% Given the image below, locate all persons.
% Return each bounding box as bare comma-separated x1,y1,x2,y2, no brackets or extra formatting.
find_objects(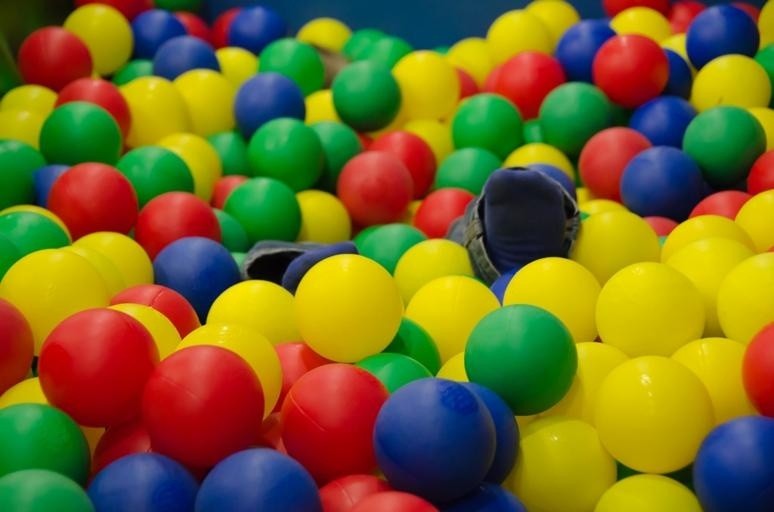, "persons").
242,166,581,289
